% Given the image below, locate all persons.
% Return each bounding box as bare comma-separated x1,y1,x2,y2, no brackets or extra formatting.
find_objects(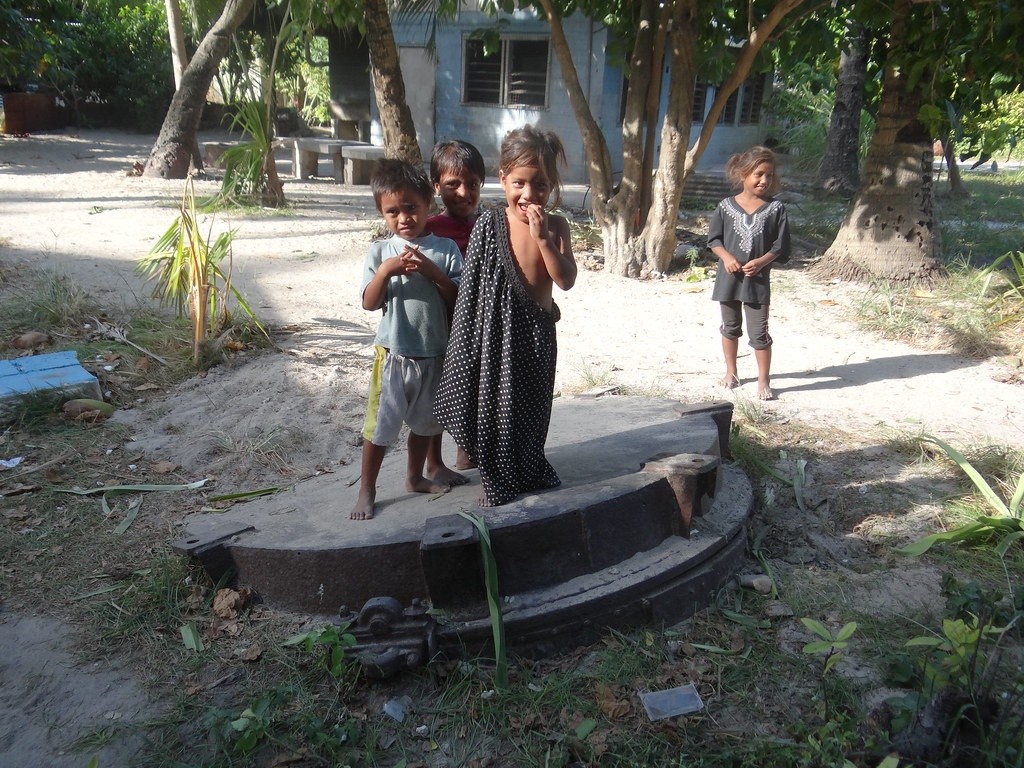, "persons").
431,124,578,506
347,138,487,520
706,146,791,401
